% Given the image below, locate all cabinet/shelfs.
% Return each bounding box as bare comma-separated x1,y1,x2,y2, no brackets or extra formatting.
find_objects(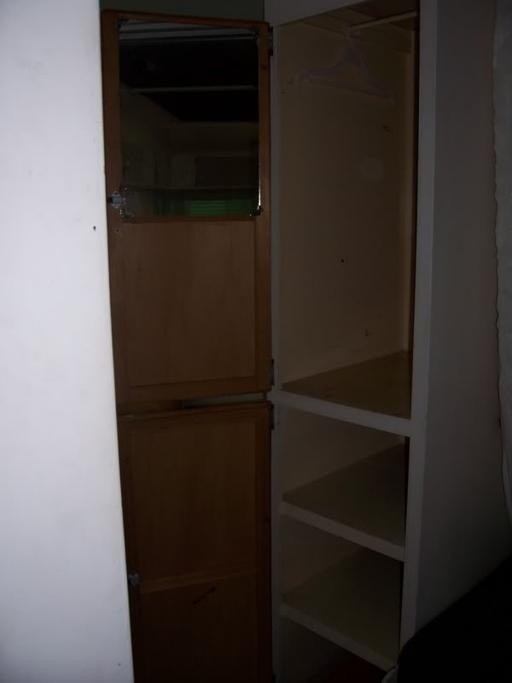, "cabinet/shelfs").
100,19,497,659
